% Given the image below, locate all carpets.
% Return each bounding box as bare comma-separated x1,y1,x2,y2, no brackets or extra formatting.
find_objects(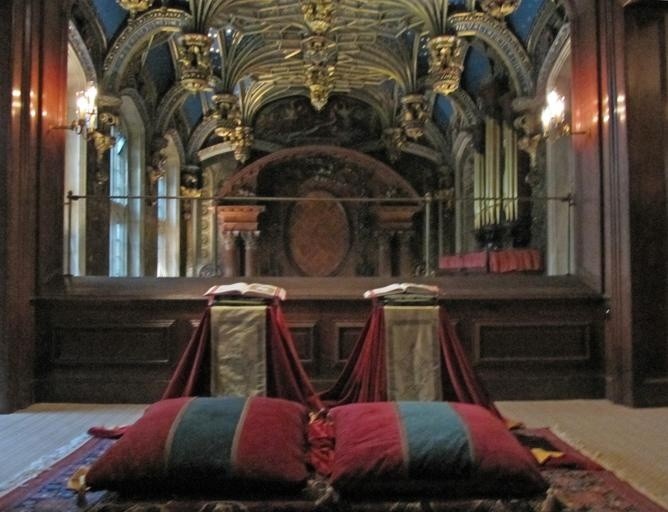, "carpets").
1,426,667,511
